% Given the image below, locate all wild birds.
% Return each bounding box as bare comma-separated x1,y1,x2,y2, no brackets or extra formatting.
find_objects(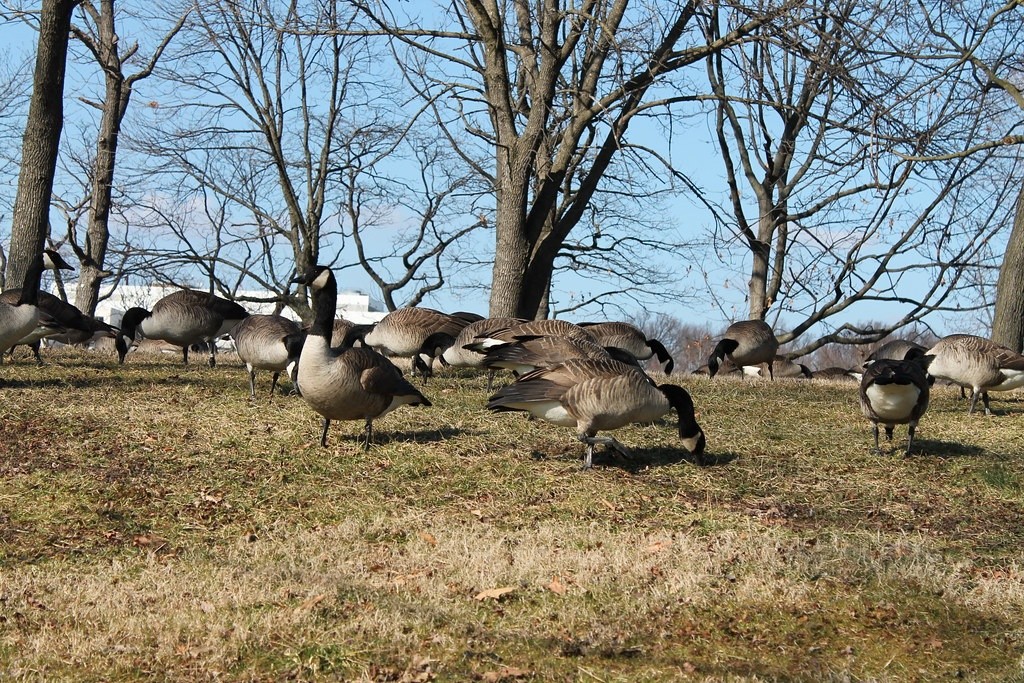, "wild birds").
40,316,122,351
0,288,140,369
0,248,76,366
689,338,937,389
926,336,1024,417
112,288,675,406
859,354,937,461
484,357,707,468
287,265,433,454
707,320,780,382
923,334,982,402
